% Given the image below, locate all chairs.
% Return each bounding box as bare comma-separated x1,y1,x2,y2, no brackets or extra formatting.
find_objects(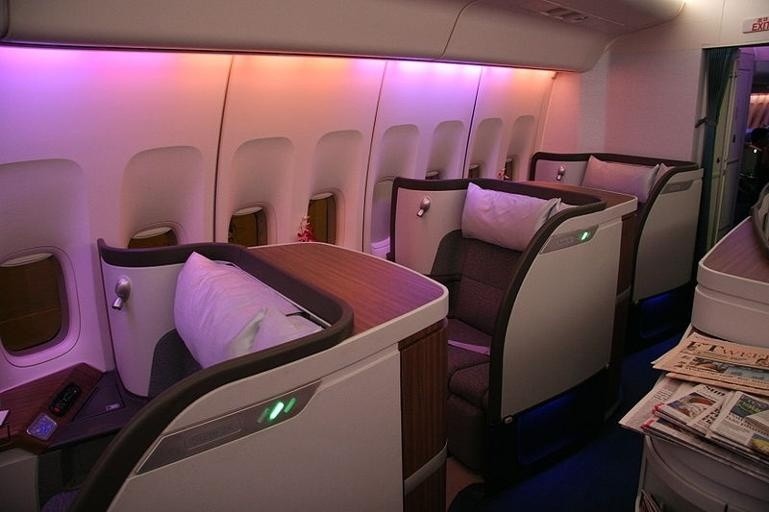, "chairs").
388,150,703,475
1,238,447,512
